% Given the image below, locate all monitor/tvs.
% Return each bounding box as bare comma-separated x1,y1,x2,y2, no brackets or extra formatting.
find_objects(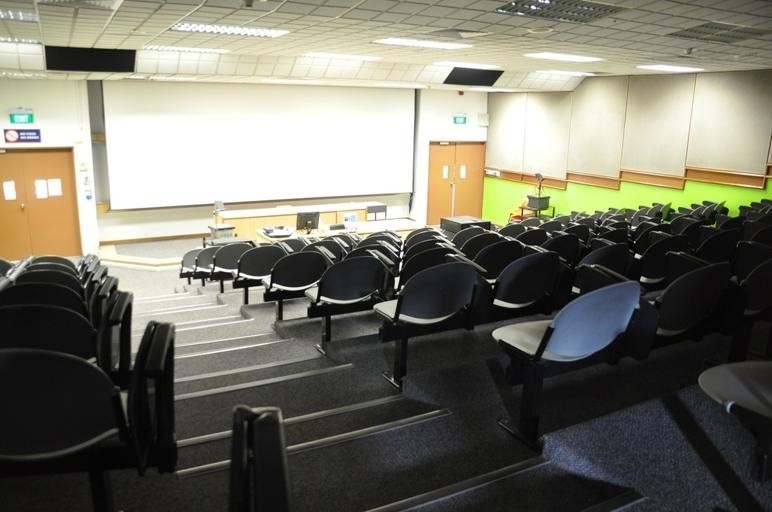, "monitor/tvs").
297,212,320,234
